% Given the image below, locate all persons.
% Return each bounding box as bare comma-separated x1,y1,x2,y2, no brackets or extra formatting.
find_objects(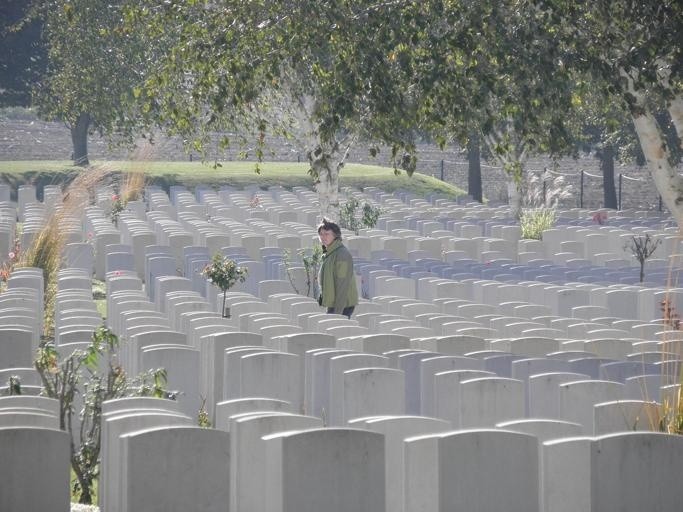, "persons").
317,221,358,319
